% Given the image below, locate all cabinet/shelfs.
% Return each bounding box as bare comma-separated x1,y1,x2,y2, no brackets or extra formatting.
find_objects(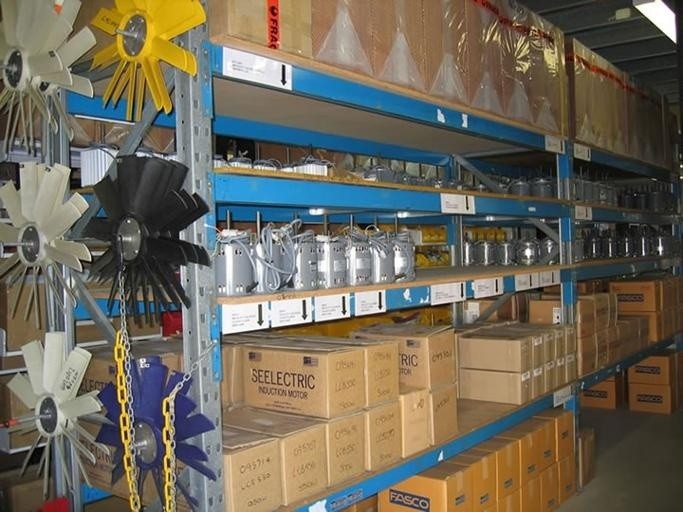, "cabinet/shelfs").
0,0,682,512
